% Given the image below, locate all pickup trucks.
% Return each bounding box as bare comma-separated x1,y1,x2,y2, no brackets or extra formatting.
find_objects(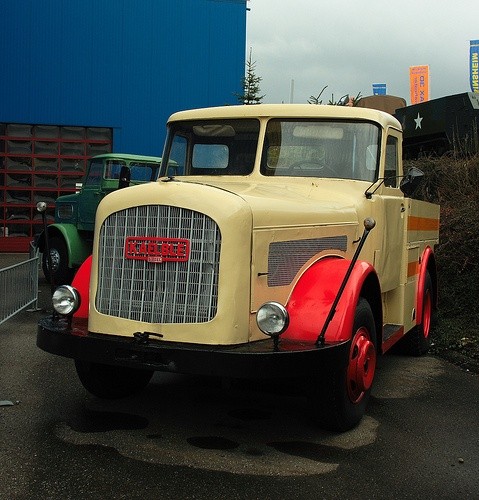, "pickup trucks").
37,104,441,435
36,153,177,288
